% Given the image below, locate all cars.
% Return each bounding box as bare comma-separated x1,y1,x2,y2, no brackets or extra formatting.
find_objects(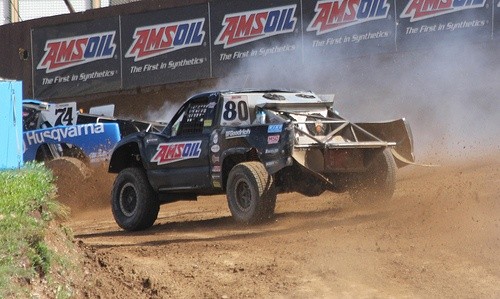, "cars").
108,88,440,233
